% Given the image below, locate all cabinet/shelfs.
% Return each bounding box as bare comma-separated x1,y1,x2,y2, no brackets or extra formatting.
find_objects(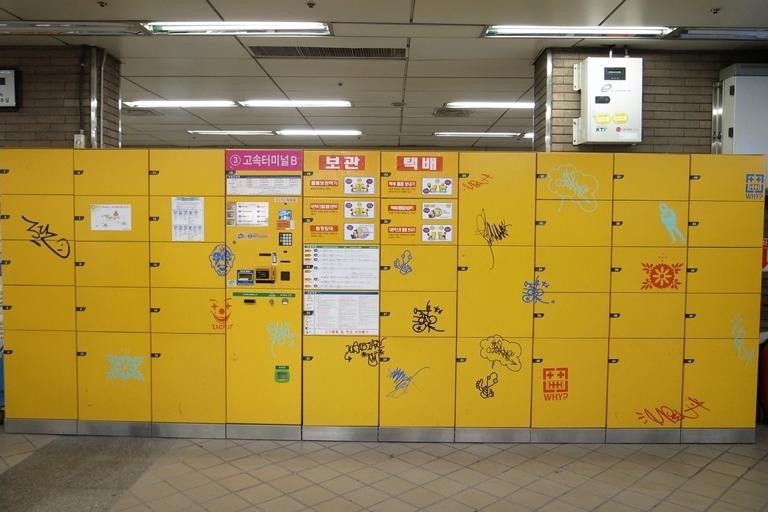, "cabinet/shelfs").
0,145,767,443
